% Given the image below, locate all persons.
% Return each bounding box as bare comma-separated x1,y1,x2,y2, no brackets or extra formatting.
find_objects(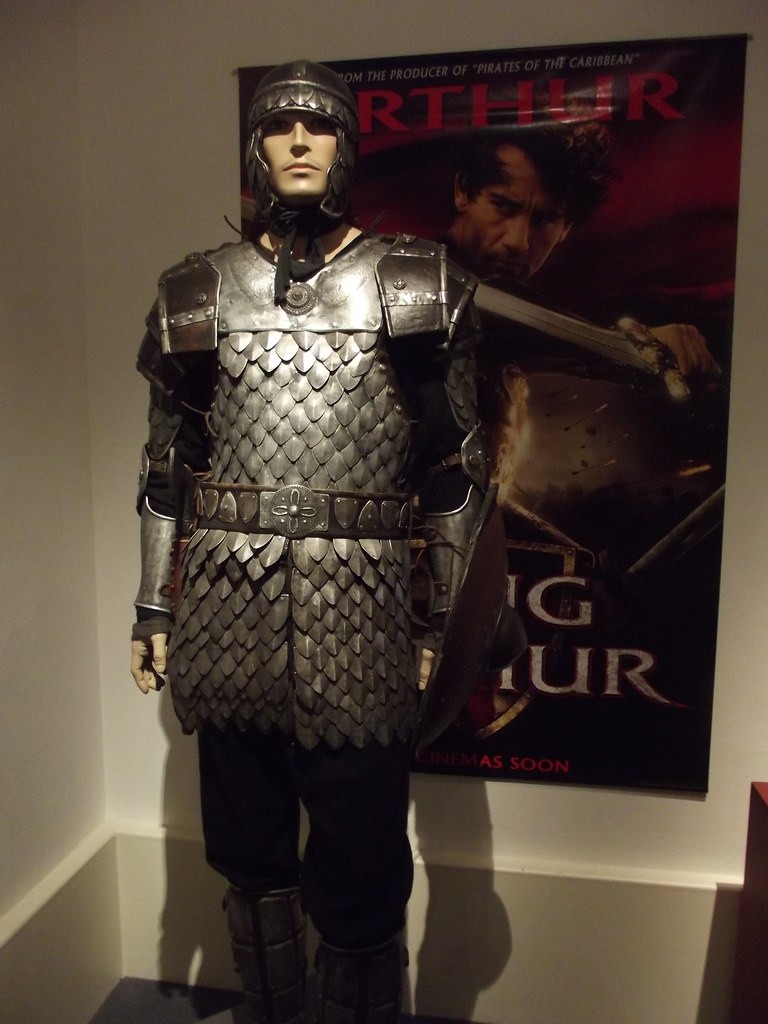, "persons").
426,106,714,776
128,59,493,1024
